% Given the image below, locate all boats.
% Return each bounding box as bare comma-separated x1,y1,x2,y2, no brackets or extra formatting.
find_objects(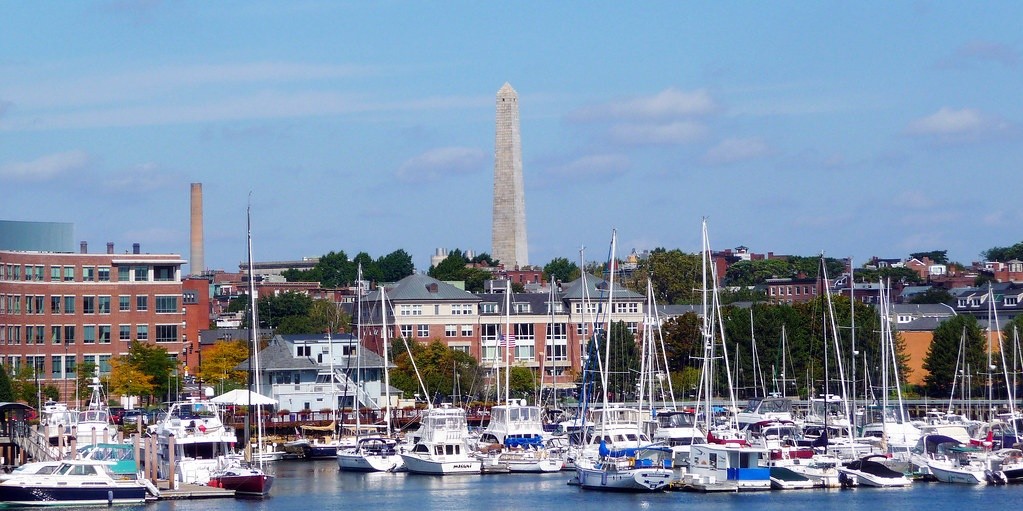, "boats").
286,216,1023,492
40,374,144,460
0,447,158,506
124,398,275,500
